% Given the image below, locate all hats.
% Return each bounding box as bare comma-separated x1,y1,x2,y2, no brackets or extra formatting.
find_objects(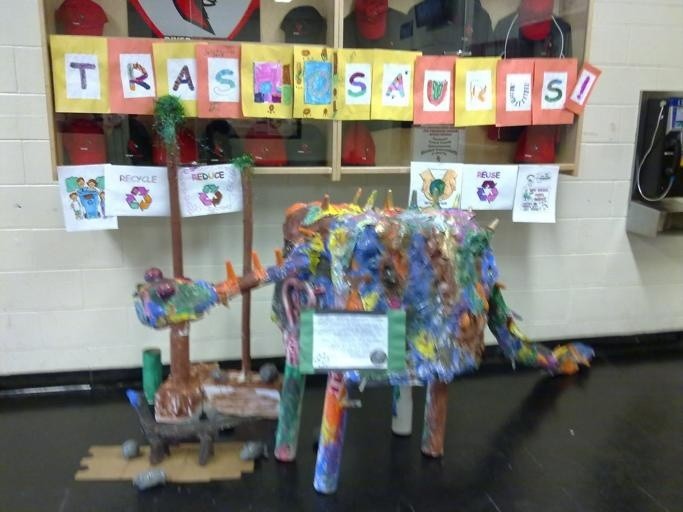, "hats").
63,120,327,166
519,1,553,40
355,0,388,40
342,124,375,165
513,126,556,162
280,6,327,44
55,0,108,36
